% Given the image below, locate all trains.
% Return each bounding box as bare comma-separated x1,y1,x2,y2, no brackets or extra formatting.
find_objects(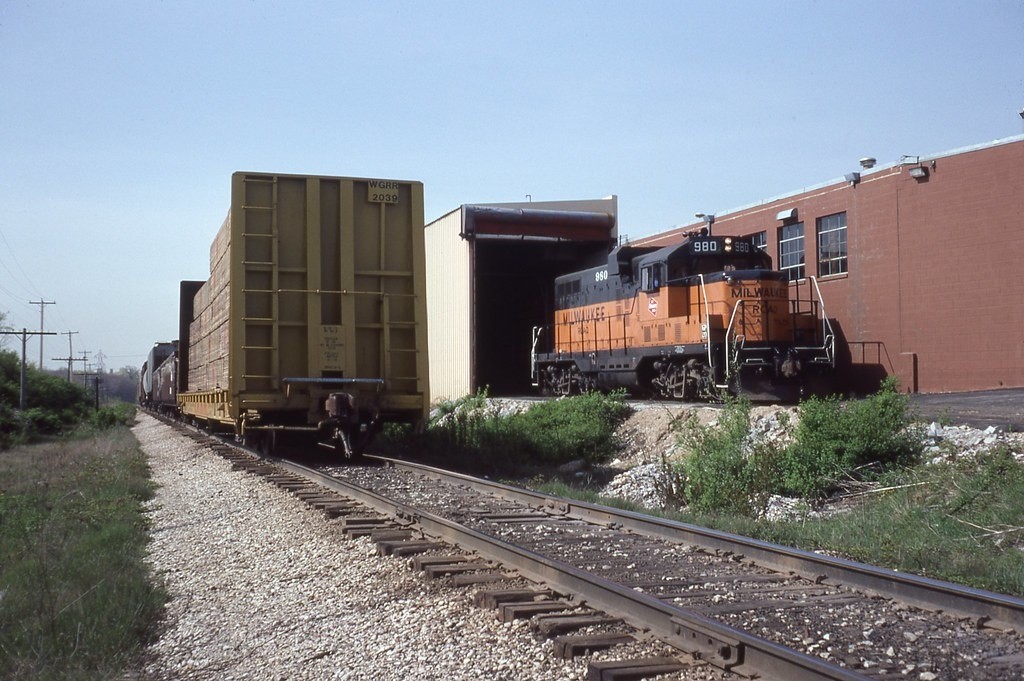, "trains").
136,170,431,460
530,226,801,408
